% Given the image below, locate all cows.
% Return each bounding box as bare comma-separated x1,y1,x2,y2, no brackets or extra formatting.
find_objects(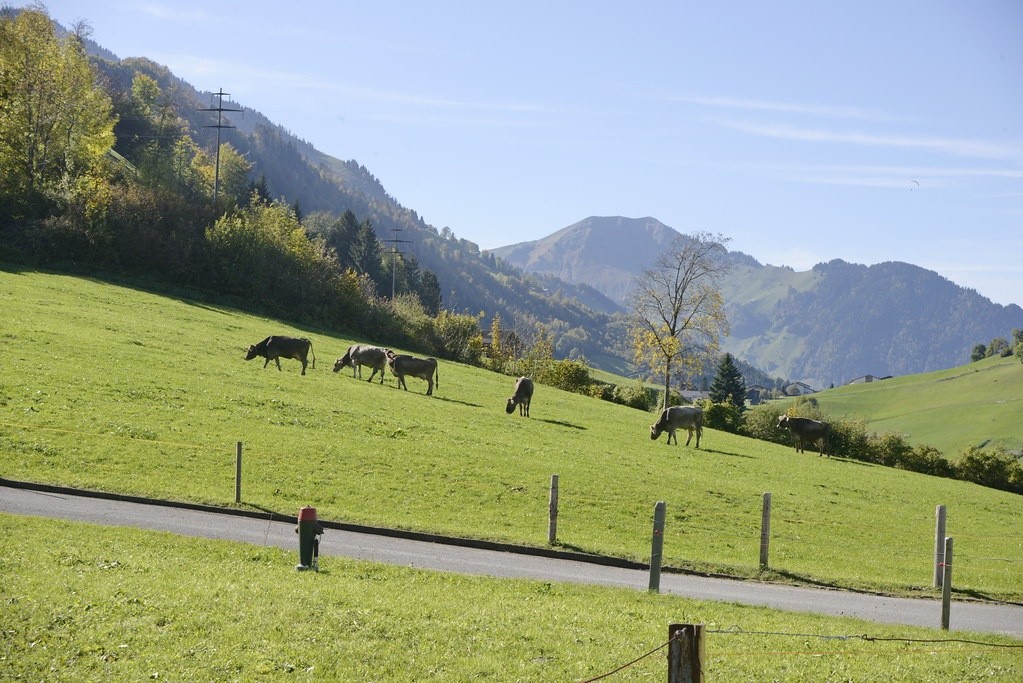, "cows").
332,344,439,397
776,415,831,459
650,406,704,449
505,374,534,418
243,335,315,376
